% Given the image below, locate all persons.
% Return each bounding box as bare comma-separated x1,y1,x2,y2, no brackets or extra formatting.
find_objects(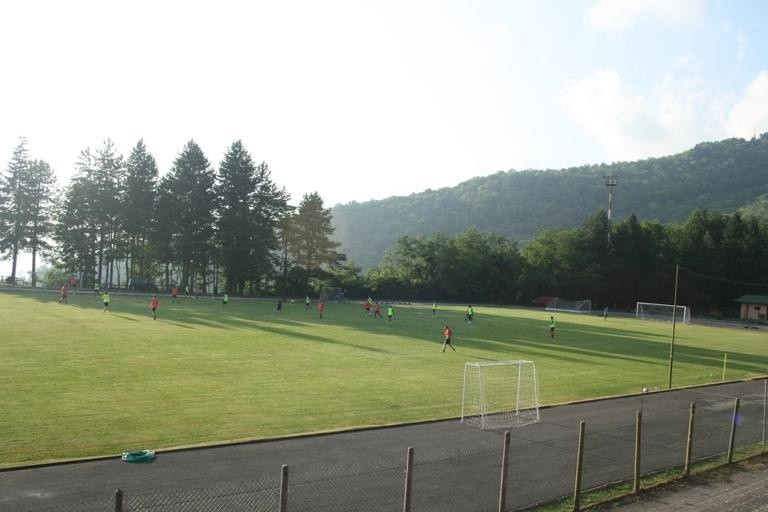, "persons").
431,299,438,319
274,297,284,318
387,304,395,323
94,281,112,314
56,275,78,305
363,294,383,319
222,292,228,311
169,284,203,305
304,295,323,319
464,303,474,322
149,296,160,322
440,324,457,353
600,303,608,322
548,316,556,339
